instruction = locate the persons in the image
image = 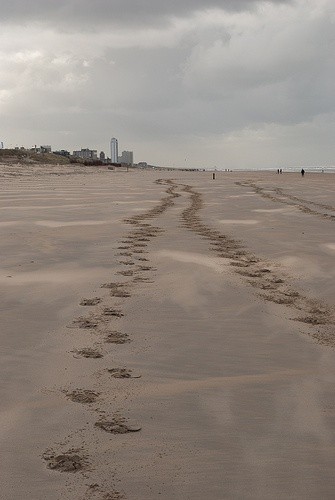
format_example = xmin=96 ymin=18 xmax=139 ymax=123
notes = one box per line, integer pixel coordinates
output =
xmin=301 ymin=169 xmax=306 ymax=178
